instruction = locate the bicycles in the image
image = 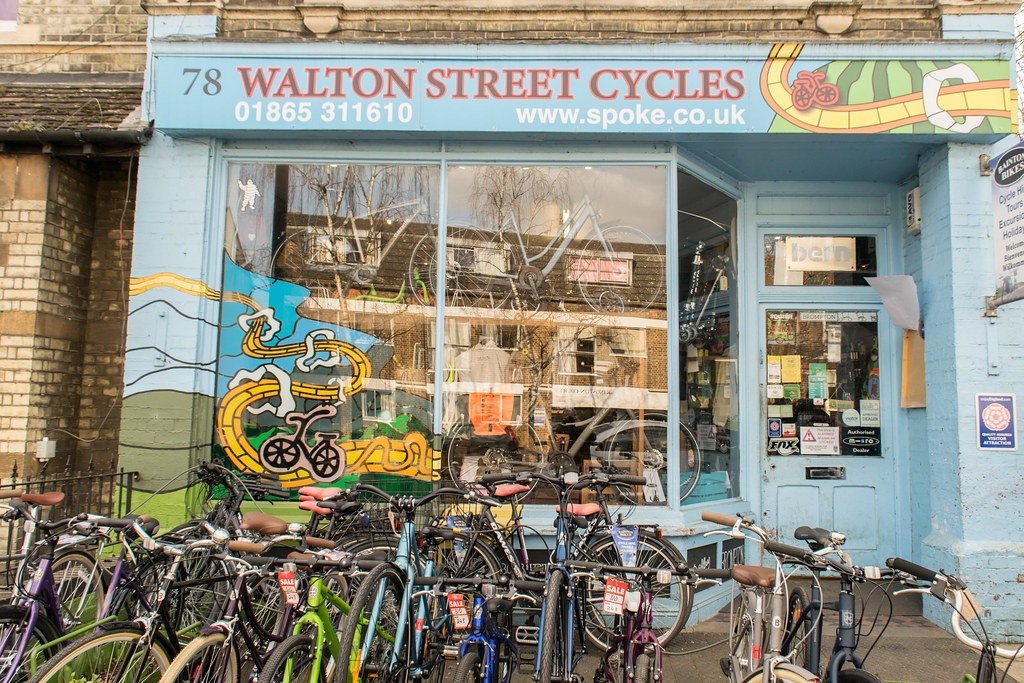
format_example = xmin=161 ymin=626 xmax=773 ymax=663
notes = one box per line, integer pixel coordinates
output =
xmin=408 ymin=163 xmax=664 ymax=316
xmin=0 ymin=455 xmax=1024 ymax=683
xmin=437 ymin=351 xmax=701 ymax=508
xmin=268 ymin=162 xmax=492 ymax=311
